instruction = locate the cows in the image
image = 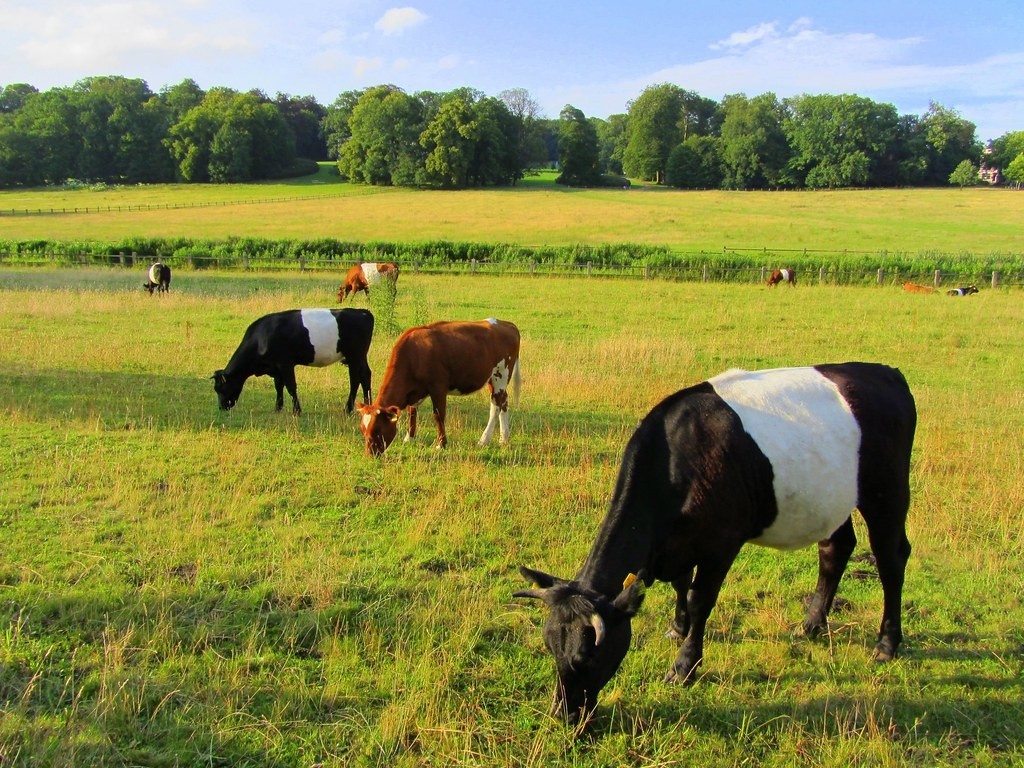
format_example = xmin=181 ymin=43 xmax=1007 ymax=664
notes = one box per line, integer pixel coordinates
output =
xmin=945 ymin=285 xmax=979 ymax=297
xmin=508 ymin=360 xmax=916 ymax=749
xmin=336 ymin=263 xmax=399 ymax=303
xmin=141 ymin=263 xmax=171 ymax=297
xmin=764 ymin=269 xmax=796 ymax=288
xmin=901 ymin=281 xmax=940 ymax=297
xmin=356 ymin=317 xmax=522 ymax=461
xmin=208 ymin=308 xmax=374 ymax=419
